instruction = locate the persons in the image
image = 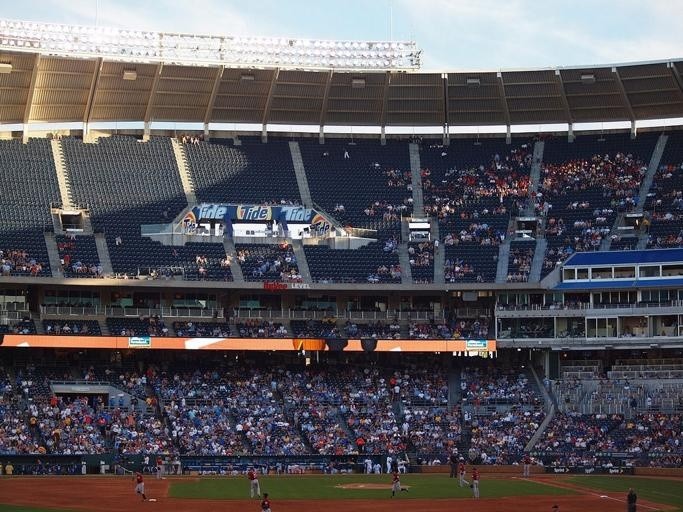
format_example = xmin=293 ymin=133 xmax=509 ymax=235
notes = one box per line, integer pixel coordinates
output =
xmin=1 ymin=141 xmax=683 ymax=283
xmin=0 ymin=353 xmax=683 ymax=512
xmin=0 ymin=302 xmax=668 ymax=340
